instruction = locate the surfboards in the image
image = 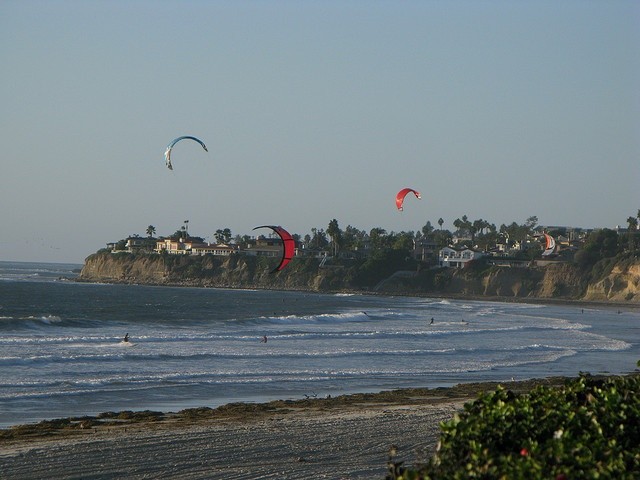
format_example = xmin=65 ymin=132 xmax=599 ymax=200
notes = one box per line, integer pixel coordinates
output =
xmin=130 ymin=343 xmax=139 ymax=347
